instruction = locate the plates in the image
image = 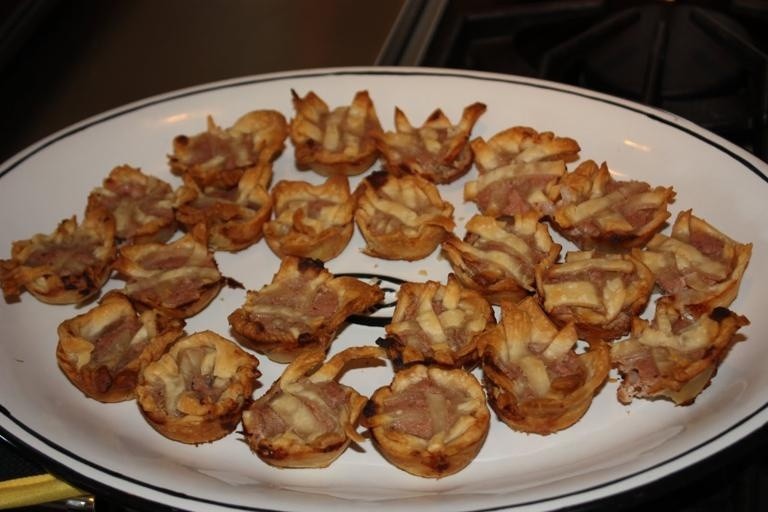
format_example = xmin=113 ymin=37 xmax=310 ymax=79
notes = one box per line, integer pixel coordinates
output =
xmin=0 ymin=67 xmax=767 ymax=512
